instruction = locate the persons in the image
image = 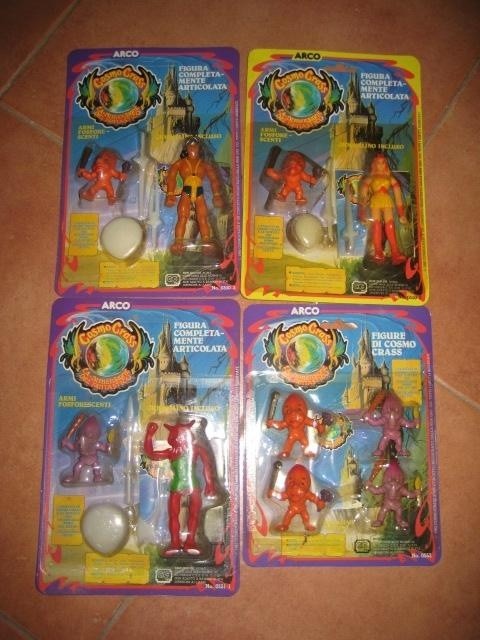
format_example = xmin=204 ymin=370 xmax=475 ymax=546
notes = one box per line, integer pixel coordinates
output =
xmin=264 ymin=393 xmax=332 ymax=458
xmin=144 ymin=419 xmax=221 ymax=557
xmin=265 ymin=144 xmax=322 ymax=207
xmin=267 ymin=460 xmax=328 ymax=534
xmin=162 ymin=135 xmax=229 ymax=256
xmin=73 ymin=142 xmax=132 ymax=207
xmin=60 ymin=409 xmax=118 ymax=487
xmin=360 ymin=389 xmax=422 ymax=455
xmin=356 ymin=150 xmax=409 ymax=265
xmin=363 ymin=454 xmax=425 ymax=527
